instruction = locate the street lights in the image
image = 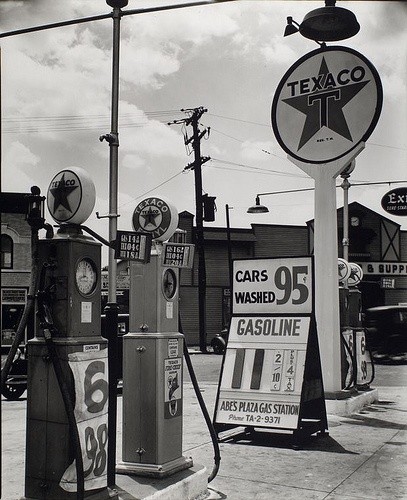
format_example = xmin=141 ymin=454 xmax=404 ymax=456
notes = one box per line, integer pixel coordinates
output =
xmin=247 ymin=184 xmax=350 ymax=323
